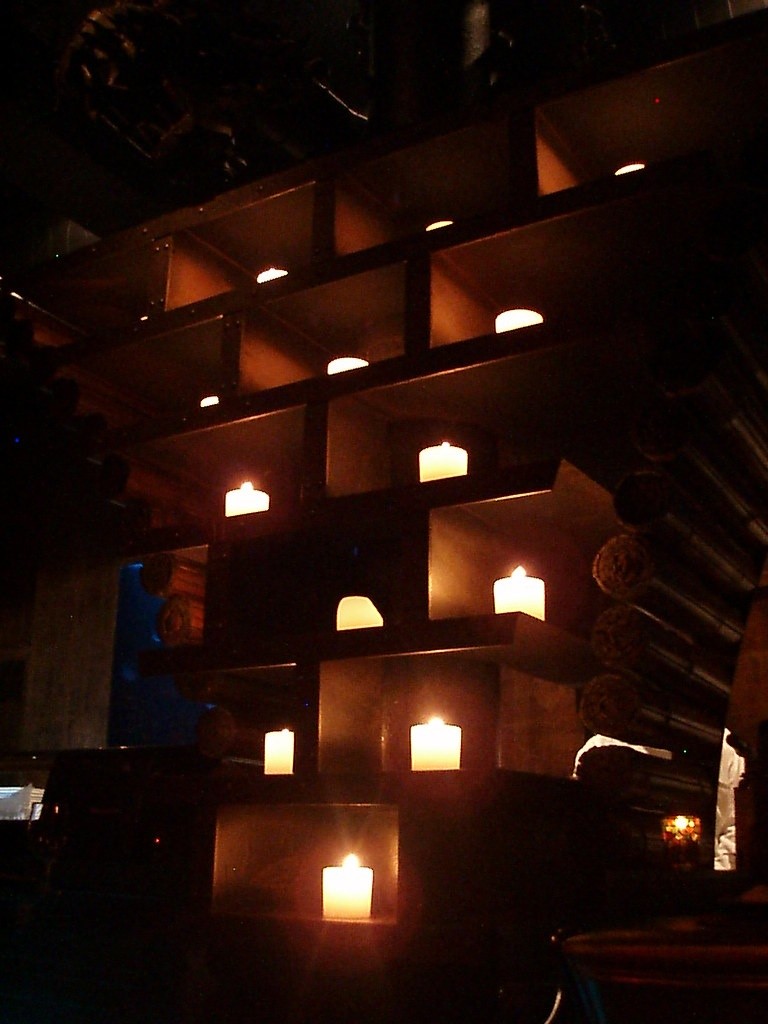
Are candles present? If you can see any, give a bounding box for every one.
[494,565,545,621]
[257,269,288,283]
[329,358,368,376]
[336,597,384,631]
[264,729,294,775]
[495,309,544,334]
[323,853,374,922]
[226,480,269,517]
[419,441,468,481]
[412,716,462,770]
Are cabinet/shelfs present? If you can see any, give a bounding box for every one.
[0,0,768,1024]
